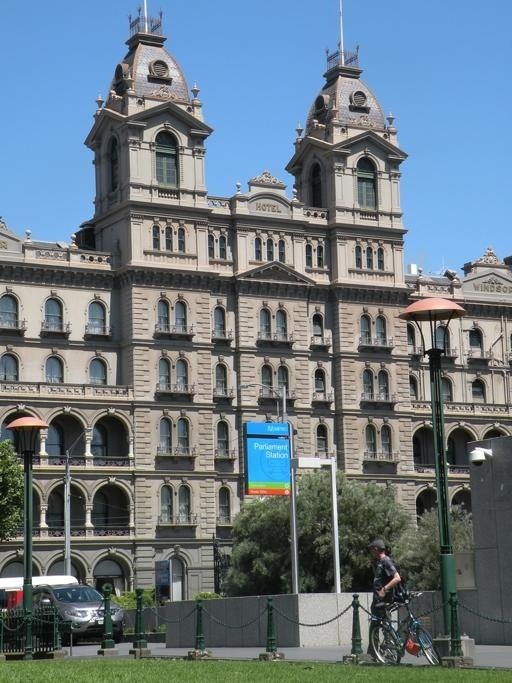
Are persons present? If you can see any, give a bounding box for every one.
[363,537,403,663]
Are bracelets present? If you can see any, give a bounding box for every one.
[383,586,388,592]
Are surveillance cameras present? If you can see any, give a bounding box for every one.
[470,446,486,467]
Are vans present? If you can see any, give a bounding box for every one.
[1,575,78,611]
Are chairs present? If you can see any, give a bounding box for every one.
[59,590,84,602]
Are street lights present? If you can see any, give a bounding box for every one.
[399,298,468,655]
[6,417,49,659]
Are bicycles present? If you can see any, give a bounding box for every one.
[369,592,442,666]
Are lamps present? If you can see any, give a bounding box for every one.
[468,447,494,467]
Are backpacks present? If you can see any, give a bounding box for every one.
[384,575,410,603]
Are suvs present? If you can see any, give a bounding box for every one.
[32,584,123,643]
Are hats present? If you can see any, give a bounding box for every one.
[367,539,386,550]
